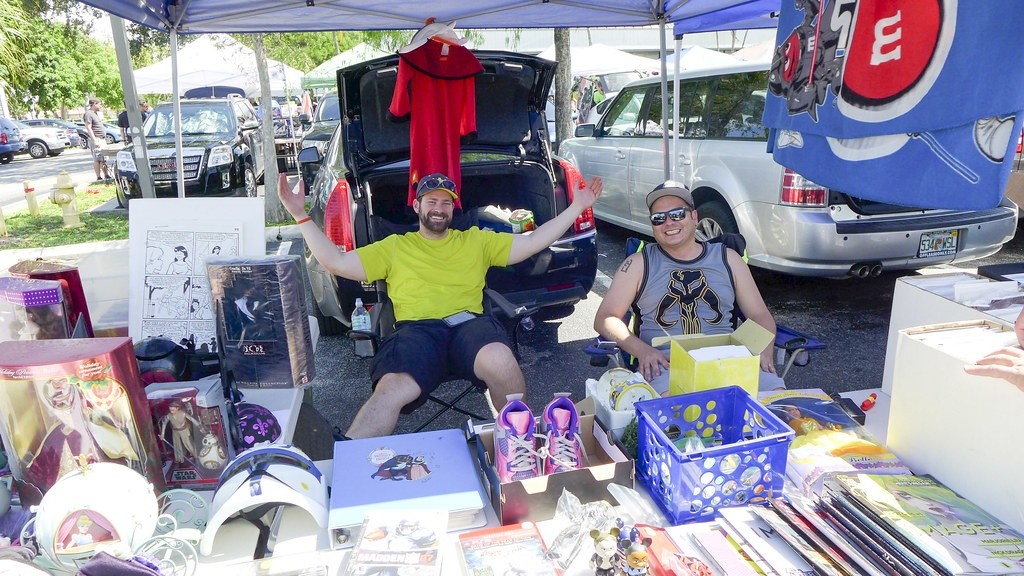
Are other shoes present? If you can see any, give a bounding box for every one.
[97,177,102,180]
[105,175,111,178]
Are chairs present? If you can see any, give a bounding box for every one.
[584,233,829,380]
[669,92,703,137]
[369,207,539,433]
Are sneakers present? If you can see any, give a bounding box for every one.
[539,392,584,477]
[492,393,539,484]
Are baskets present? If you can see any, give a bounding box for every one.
[633,386,795,526]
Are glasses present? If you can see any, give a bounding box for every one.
[649,207,692,225]
[417,178,456,192]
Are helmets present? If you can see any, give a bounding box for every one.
[131,337,191,381]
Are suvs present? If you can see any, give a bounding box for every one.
[112,86,265,208]
[559,58,1018,281]
[298,89,340,195]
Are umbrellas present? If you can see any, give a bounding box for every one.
[120,32,397,102]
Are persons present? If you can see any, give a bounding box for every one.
[117,98,153,145]
[782,404,837,437]
[964,307,1024,396]
[277,174,602,439]
[83,98,112,180]
[589,518,651,576]
[594,180,787,396]
[11,304,66,341]
[160,403,208,465]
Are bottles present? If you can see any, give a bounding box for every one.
[351,298,375,357]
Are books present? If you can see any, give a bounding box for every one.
[691,473,1024,576]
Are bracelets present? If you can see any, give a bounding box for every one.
[297,216,312,224]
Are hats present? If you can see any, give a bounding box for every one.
[646,180,694,211]
[417,173,458,200]
[87,99,101,106]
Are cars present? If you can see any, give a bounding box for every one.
[0,115,128,164]
[302,49,599,336]
[545,66,648,153]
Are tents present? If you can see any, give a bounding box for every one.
[533,41,778,104]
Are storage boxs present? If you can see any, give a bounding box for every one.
[8,261,95,341]
[463,395,635,526]
[0,276,70,339]
[886,318,1024,534]
[144,378,237,490]
[636,384,796,525]
[978,262,1024,287]
[652,318,776,437]
[0,338,171,514]
[880,272,1024,397]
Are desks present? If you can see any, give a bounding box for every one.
[145,316,891,575]
